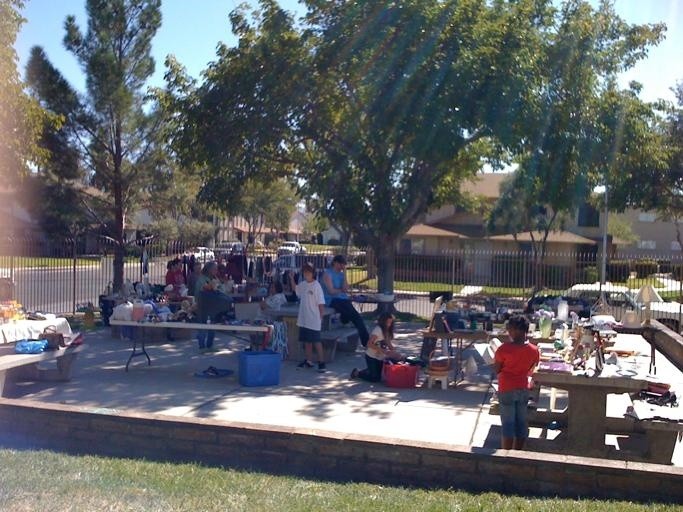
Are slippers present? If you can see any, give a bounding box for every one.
[351,368,359,379]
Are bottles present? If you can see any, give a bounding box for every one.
[557,300,569,322]
[132,298,144,321]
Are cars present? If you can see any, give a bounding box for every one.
[446,285,507,323]
[178,241,335,285]
[523,284,681,333]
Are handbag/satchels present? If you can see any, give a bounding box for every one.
[38,324,65,350]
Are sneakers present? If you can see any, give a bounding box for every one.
[298,359,315,367]
[318,362,326,373]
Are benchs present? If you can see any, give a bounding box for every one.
[1,342,89,394]
[628,389,681,464]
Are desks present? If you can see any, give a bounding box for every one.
[0,318,72,348]
[417,308,645,456]
[109,287,400,373]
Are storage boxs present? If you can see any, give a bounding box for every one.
[383,361,421,388]
[239,350,281,387]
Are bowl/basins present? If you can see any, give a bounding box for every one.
[375,293,394,303]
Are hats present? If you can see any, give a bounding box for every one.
[334,255,347,265]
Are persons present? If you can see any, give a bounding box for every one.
[494,315,540,449]
[286,261,326,373]
[166,257,224,354]
[320,255,369,347]
[348,313,405,382]
[254,280,286,320]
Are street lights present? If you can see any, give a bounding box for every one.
[594,176,609,306]
